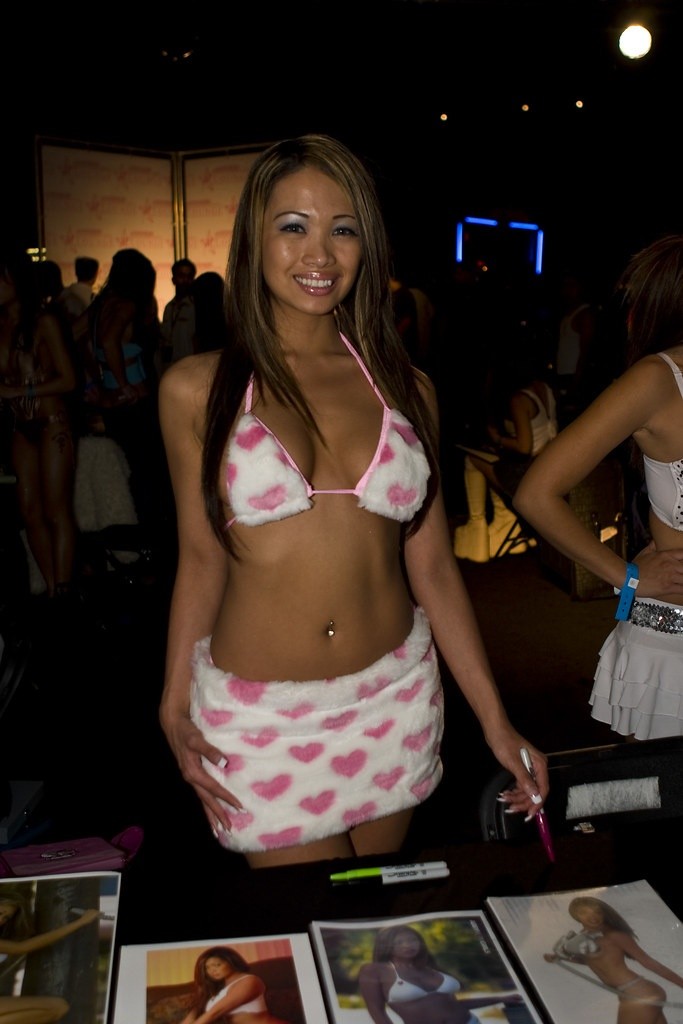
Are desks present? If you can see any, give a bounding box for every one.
[101,818,683,1024]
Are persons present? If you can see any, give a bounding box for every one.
[0,890,104,1024]
[159,133,551,869]
[0,247,226,611]
[513,340,682,744]
[179,947,289,1024]
[378,236,683,565]
[359,925,481,1024]
[544,897,683,1024]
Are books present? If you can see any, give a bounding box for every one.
[0,871,122,1024]
[113,932,329,1024]
[309,909,545,1024]
[483,879,683,1024]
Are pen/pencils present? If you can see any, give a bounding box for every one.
[325,859,451,883]
[520,745,557,869]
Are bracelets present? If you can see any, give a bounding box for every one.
[613,563,639,622]
[28,385,32,398]
